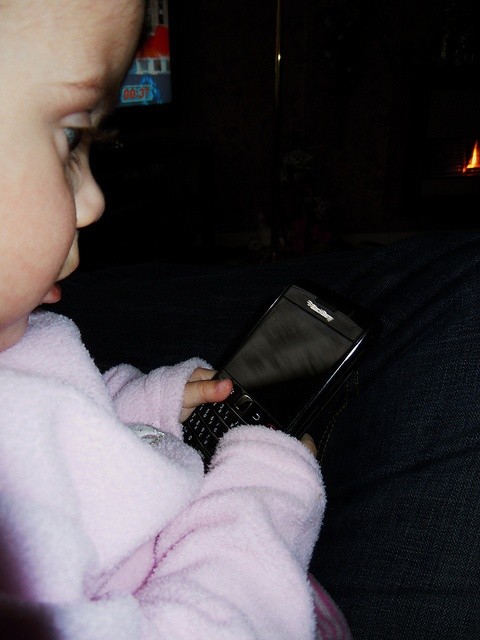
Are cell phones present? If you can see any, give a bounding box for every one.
[181,283,369,475]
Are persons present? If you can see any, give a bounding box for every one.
[1,1,348,638]
